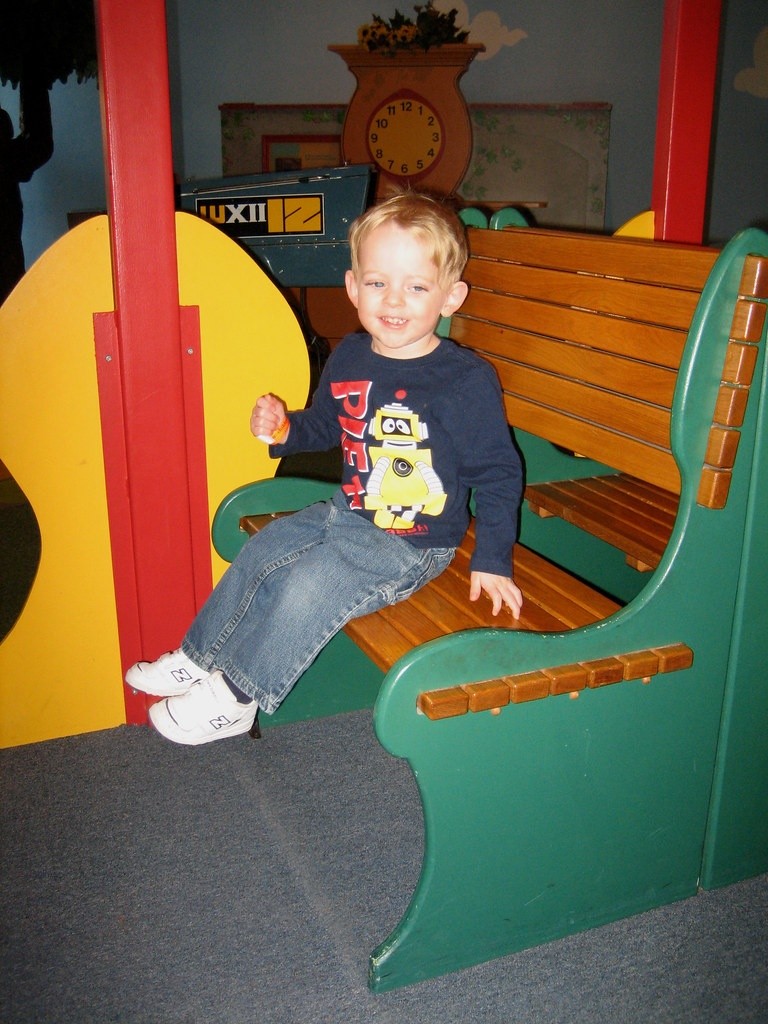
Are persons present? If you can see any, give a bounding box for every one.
[125,186,527,747]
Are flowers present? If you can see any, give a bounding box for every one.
[353,0,471,56]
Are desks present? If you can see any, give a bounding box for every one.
[66,200,548,407]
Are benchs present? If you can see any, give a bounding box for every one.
[211,203,768,996]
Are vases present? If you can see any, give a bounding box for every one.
[326,43,486,203]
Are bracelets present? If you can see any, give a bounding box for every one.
[270,415,290,445]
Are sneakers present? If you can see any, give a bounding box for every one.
[148,669,259,746]
[124,646,212,697]
